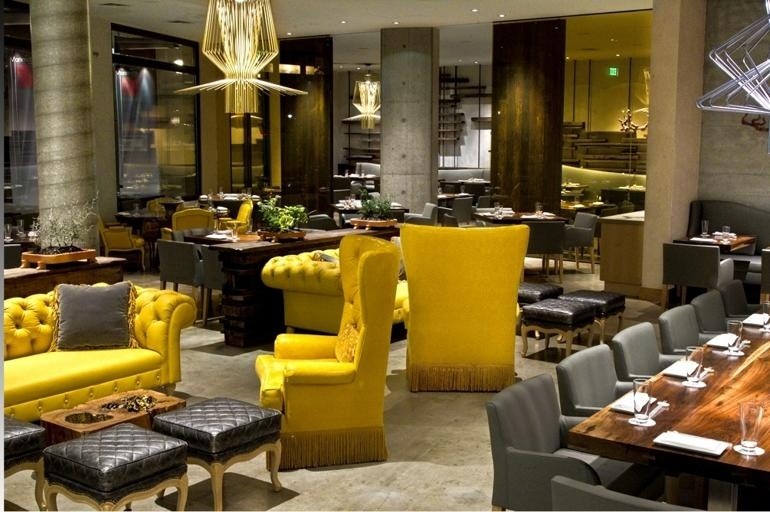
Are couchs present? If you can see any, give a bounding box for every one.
[689,198,769,284]
[261,249,410,334]
[1,281,198,424]
[562,164,646,248]
[355,162,490,187]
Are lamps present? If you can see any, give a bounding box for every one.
[339,75,382,129]
[173,0,310,116]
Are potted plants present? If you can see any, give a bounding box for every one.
[350,187,397,230]
[257,194,316,242]
[20,191,102,270]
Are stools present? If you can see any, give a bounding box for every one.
[151,397,284,511]
[4,412,48,512]
[519,298,601,359]
[516,281,563,339]
[40,421,189,512]
[557,290,624,345]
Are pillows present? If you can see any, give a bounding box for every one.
[390,234,407,277]
[51,279,135,354]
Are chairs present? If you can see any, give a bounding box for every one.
[438,177,446,185]
[200,244,227,326]
[484,372,634,511]
[439,197,473,224]
[658,305,718,357]
[333,188,351,204]
[254,236,403,473]
[565,211,599,276]
[549,475,698,512]
[156,239,212,323]
[612,322,687,380]
[690,287,742,335]
[554,342,644,417]
[3,243,20,269]
[172,230,186,242]
[662,241,734,304]
[442,179,461,194]
[561,199,604,212]
[192,227,213,237]
[146,196,185,212]
[215,197,253,236]
[520,220,567,284]
[471,196,492,216]
[464,182,486,203]
[442,214,459,229]
[97,214,147,272]
[298,215,338,231]
[161,208,215,291]
[593,203,619,257]
[399,223,530,392]
[619,200,635,214]
[715,279,763,318]
[403,202,439,226]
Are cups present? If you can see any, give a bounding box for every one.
[722,225,731,244]
[350,194,355,203]
[762,303,770,330]
[133,203,140,217]
[740,402,764,451]
[242,188,247,194]
[701,220,709,236]
[497,204,504,220]
[247,187,252,196]
[208,189,213,201]
[685,346,705,385]
[218,187,224,197]
[344,196,350,207]
[633,378,650,424]
[535,202,543,218]
[5,224,12,240]
[17,219,25,234]
[726,319,743,355]
